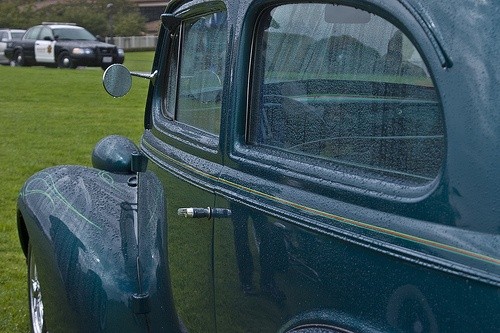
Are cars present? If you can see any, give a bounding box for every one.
[16,0,500,333]
[4,22,125,71]
[0,28,26,66]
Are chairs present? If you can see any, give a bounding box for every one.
[258,95,335,149]
